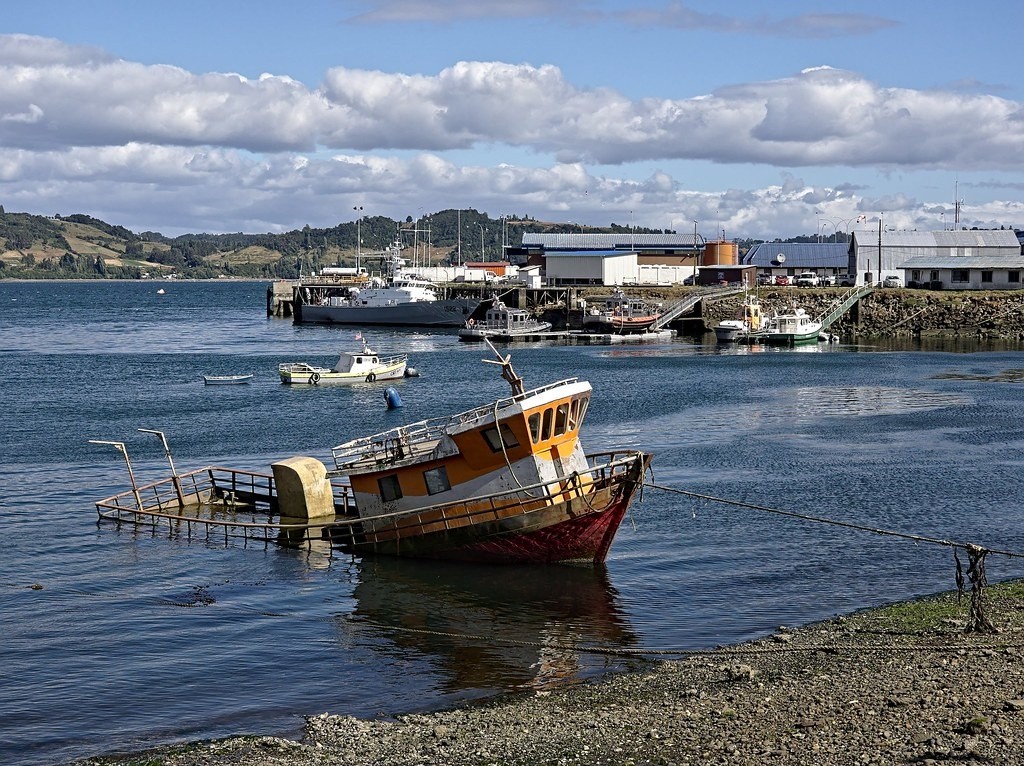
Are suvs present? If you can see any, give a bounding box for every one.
[404,273,431,282]
[798,271,817,287]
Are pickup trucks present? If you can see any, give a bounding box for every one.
[837,274,857,287]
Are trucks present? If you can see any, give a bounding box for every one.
[464,269,499,284]
[321,267,367,277]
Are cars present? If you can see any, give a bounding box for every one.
[885,276,903,287]
[792,275,802,285]
[818,276,830,287]
[492,274,519,284]
[775,275,789,286]
[757,274,772,285]
[683,274,699,286]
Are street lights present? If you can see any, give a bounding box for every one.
[415,206,433,267]
[821,216,858,243]
[941,212,947,231]
[816,211,819,243]
[501,216,509,261]
[694,220,698,285]
[353,206,363,275]
[568,221,583,234]
[458,208,465,267]
[881,212,883,230]
[474,221,484,263]
[821,224,827,244]
[716,210,720,264]
[631,210,633,252]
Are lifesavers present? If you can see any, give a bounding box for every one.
[311,373,320,382]
[743,319,750,327]
[469,319,474,324]
[368,372,376,382]
[616,306,620,312]
[280,375,287,383]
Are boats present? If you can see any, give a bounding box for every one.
[716,273,770,346]
[277,337,408,385]
[582,291,665,334]
[89,336,649,582]
[202,374,254,385]
[456,302,552,341]
[300,231,485,329]
[735,302,823,346]
[817,332,840,343]
